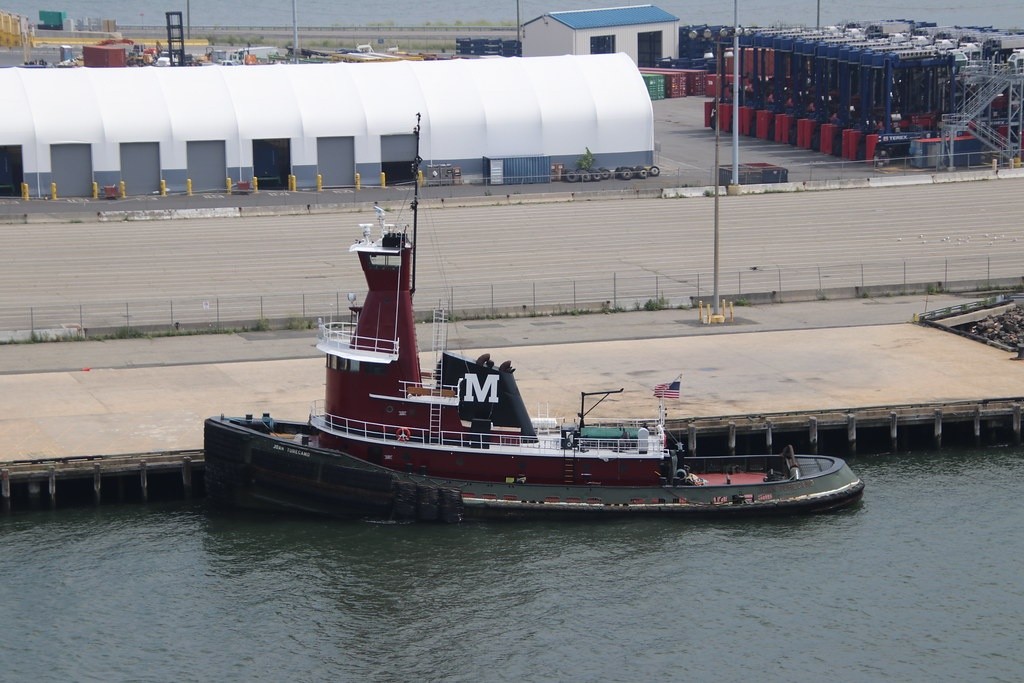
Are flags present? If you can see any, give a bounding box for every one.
[654,382,679,398]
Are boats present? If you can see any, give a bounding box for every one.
[202,109,867,525]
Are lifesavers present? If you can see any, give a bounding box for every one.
[396,428,411,441]
